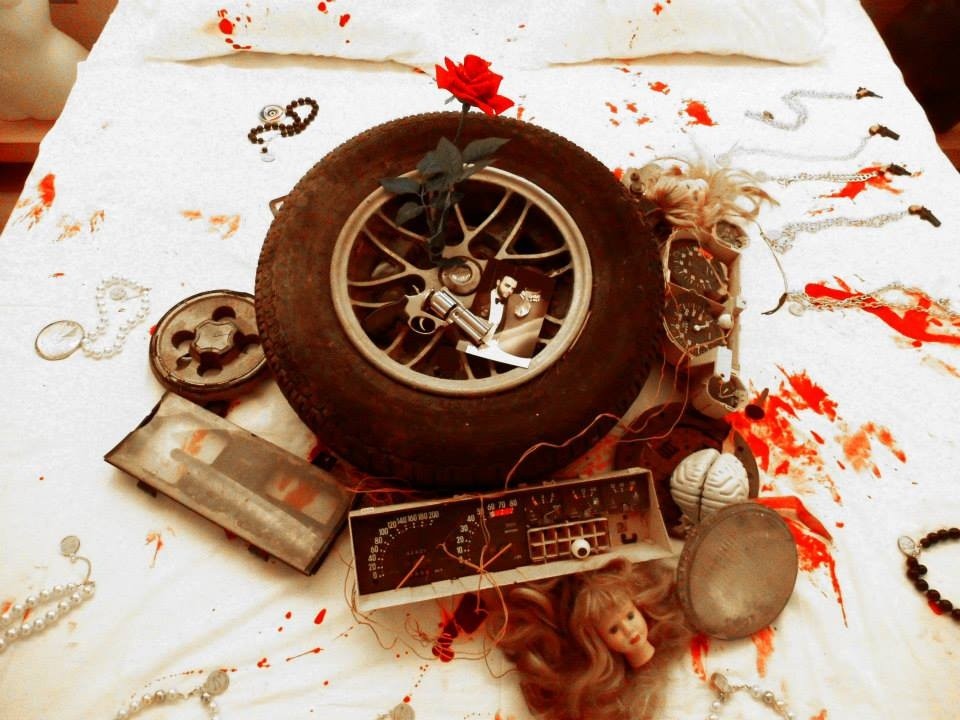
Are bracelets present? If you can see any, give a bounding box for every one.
[248,98,319,163]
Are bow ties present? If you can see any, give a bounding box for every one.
[495,298,505,304]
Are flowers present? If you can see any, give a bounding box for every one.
[386,40,517,252]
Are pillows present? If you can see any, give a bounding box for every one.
[135,0,848,63]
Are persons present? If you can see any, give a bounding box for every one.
[645,166,762,231]
[495,556,691,720]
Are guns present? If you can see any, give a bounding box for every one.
[361,283,496,345]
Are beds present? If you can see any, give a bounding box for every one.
[0,0,960,720]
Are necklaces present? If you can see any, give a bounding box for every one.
[116,670,229,720]
[897,527,960,621]
[745,87,883,132]
[785,282,957,322]
[760,205,941,256]
[0,537,95,652]
[709,672,795,720]
[35,275,149,360]
[737,123,899,162]
[775,163,912,189]
